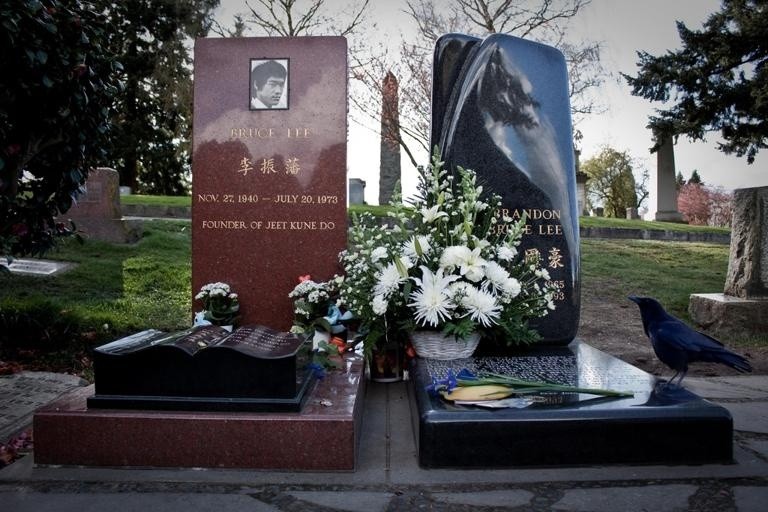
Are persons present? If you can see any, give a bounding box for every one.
[250,60,287,109]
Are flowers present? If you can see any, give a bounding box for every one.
[425,368,635,401]
[193,280,241,326]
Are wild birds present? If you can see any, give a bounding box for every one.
[625,292,752,393]
